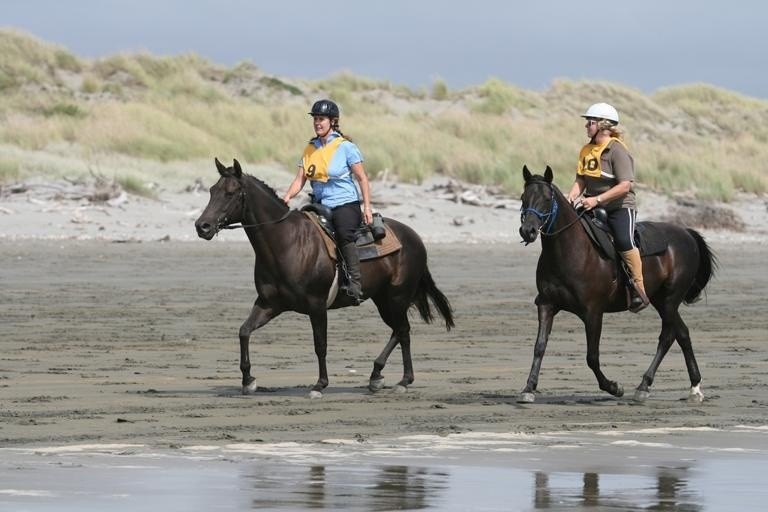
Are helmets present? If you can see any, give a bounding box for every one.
[580,103,619,125]
[308,100,339,118]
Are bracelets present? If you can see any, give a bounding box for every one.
[595,194,602,207]
[364,207,372,210]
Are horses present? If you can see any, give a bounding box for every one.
[193,156,457,400]
[515,164,719,405]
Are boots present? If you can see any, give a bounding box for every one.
[340,241,362,306]
[619,246,647,307]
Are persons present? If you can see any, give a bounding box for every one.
[566,103,649,312]
[281,99,375,307]
